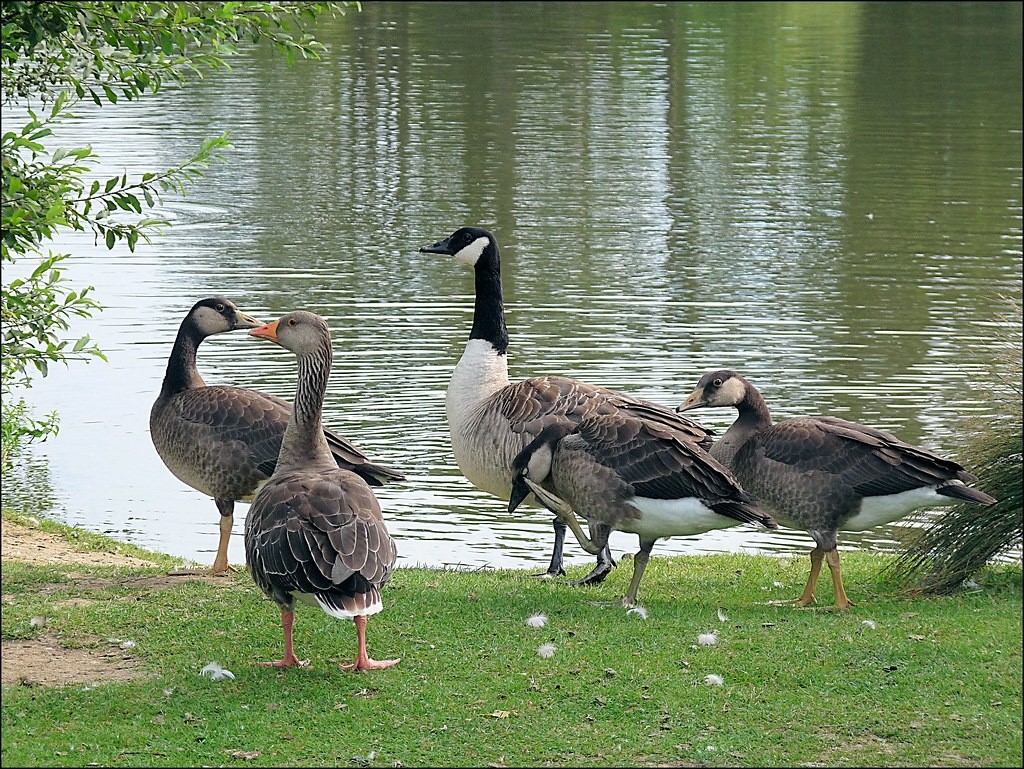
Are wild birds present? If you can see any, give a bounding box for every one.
[148,297,404,673]
[417,226,998,613]
